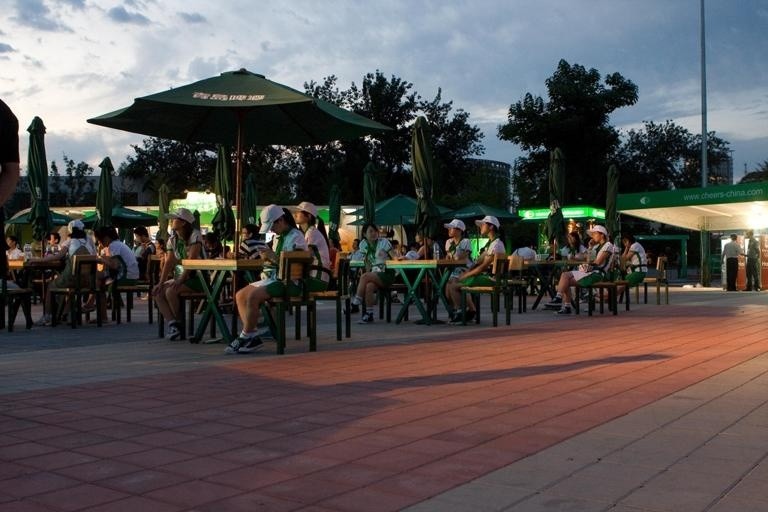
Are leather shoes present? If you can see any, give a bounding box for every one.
[742,287,752,291]
[756,288,761,292]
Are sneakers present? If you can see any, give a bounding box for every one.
[81,305,95,313]
[581,287,596,303]
[342,303,360,317]
[558,307,573,316]
[357,312,374,324]
[447,306,476,325]
[546,297,563,307]
[223,316,270,354]
[35,318,52,326]
[166,321,181,340]
[88,319,112,327]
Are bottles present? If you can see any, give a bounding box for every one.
[54,242,58,256]
[46,242,51,257]
[24,242,30,261]
[433,242,439,260]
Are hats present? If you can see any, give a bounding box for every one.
[164,208,196,224]
[475,216,501,230]
[585,225,608,239]
[259,204,284,234]
[406,251,421,260]
[444,218,467,232]
[291,202,319,219]
[68,219,86,234]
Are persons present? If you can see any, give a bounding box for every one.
[1,204,647,353]
[722,230,761,291]
